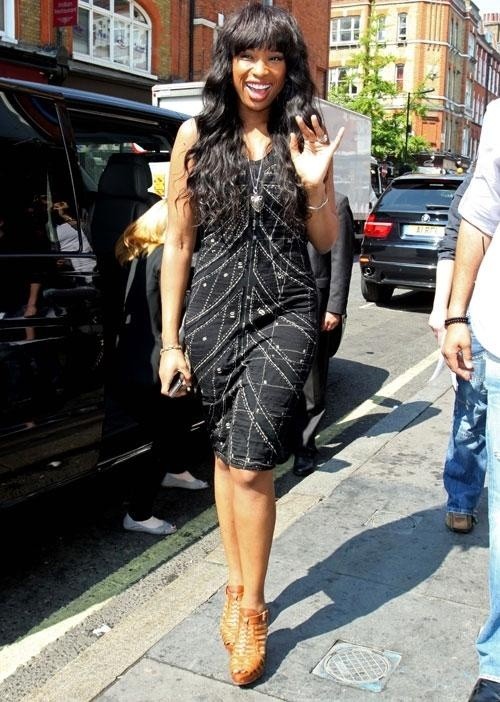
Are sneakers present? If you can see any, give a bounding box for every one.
[163,471,209,489]
[446,511,477,533]
[124,513,177,534]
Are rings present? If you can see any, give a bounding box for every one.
[316,135,329,145]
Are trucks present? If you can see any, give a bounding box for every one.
[142,78,384,251]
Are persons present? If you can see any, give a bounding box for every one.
[441,98,499,701]
[18,195,99,342]
[157,2,346,688]
[290,192,355,476]
[426,154,489,534]
[97,192,213,536]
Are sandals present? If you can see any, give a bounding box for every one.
[230,607,269,685]
[220,585,243,651]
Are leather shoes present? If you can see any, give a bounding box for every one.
[468,679,499,701]
[294,449,317,476]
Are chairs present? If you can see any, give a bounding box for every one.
[92,153,161,341]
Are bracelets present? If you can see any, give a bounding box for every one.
[445,317,469,326]
[159,346,187,355]
[302,200,332,210]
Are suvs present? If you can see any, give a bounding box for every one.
[356,169,471,303]
[0,26,346,507]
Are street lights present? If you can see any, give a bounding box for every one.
[403,88,438,160]
